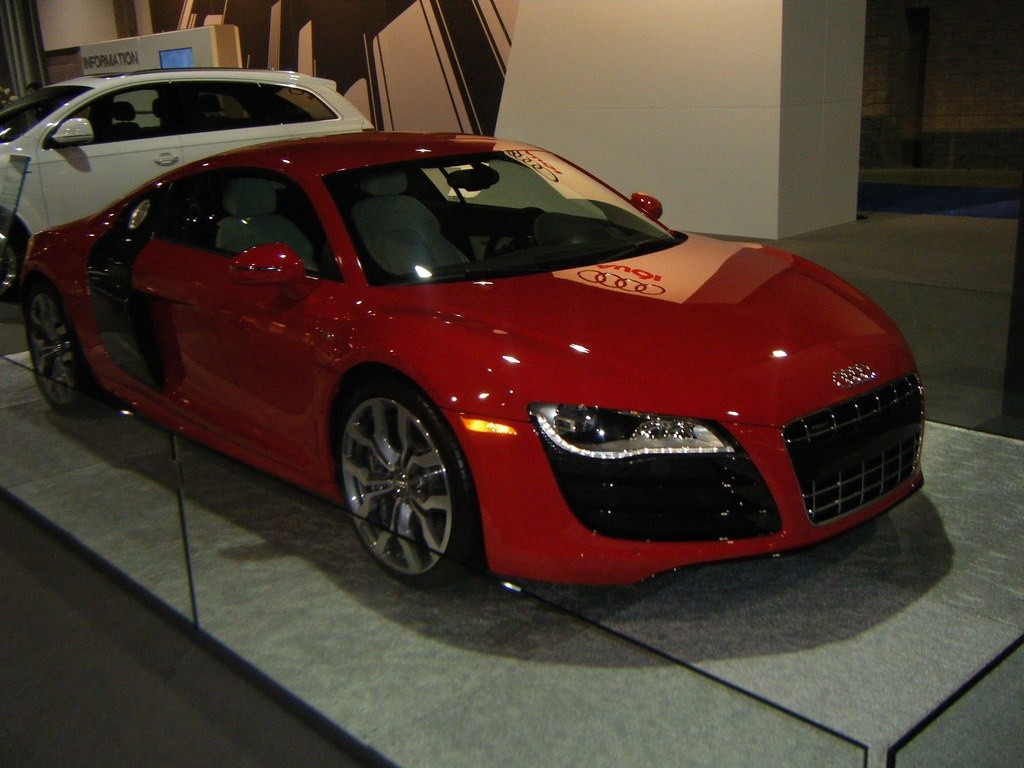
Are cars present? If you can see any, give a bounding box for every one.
[0,66,378,303]
[17,131,926,590]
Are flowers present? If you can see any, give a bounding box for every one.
[0,88,19,107]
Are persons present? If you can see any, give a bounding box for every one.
[25,82,59,122]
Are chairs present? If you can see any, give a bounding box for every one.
[110,102,139,131]
[201,94,232,126]
[215,178,317,274]
[352,174,469,280]
